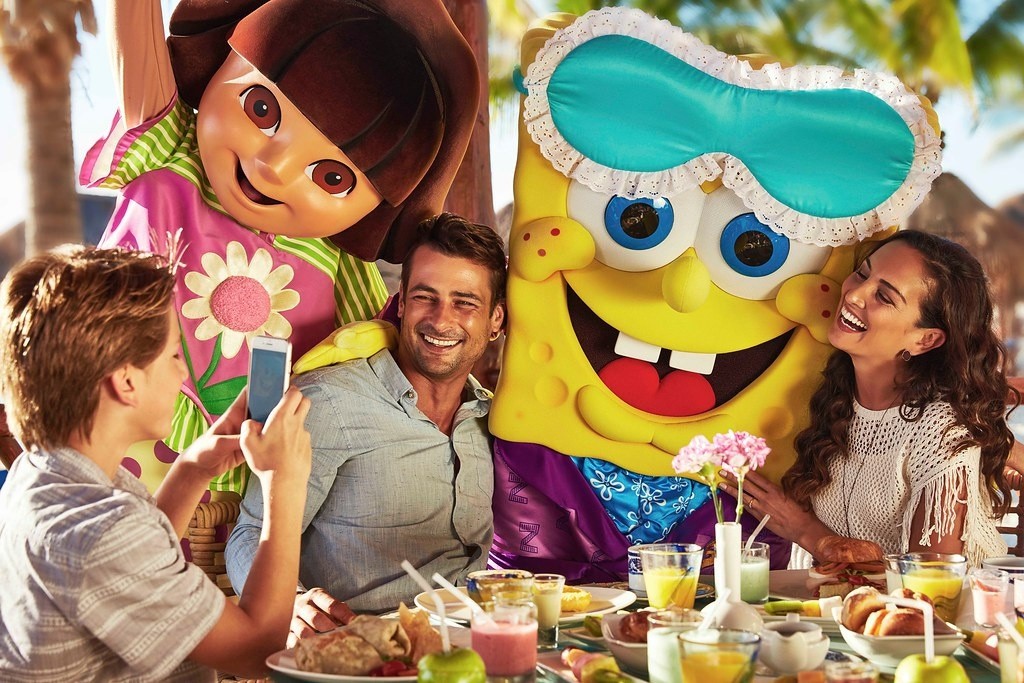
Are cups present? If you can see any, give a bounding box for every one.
[995,609,1024,683]
[882,551,969,626]
[626,545,667,591]
[969,569,1010,630]
[528,574,566,650]
[676,625,763,683]
[639,543,704,610]
[466,571,537,683]
[713,541,770,609]
[645,610,706,683]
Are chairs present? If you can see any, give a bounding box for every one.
[187,502,275,683]
[988,438,1023,559]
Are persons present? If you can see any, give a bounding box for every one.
[76,0,479,588]
[0,243,313,683]
[717,226,1019,570]
[223,211,495,643]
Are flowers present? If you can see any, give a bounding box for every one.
[671,430,772,525]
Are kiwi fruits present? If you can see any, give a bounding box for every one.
[763,600,804,616]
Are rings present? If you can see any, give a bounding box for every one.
[749,498,754,509]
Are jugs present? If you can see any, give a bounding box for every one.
[754,612,831,673]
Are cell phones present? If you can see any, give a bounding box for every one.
[244,336,292,427]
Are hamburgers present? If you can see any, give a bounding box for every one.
[806,536,889,596]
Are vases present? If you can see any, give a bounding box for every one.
[700,522,764,631]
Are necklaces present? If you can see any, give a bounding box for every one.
[842,391,904,538]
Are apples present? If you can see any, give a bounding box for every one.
[892,652,971,683]
[415,646,488,683]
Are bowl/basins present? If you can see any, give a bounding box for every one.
[601,613,647,676]
[982,557,1024,582]
[832,605,968,673]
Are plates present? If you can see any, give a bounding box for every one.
[414,583,637,625]
[755,648,879,683]
[265,646,419,683]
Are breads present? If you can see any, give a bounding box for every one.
[840,587,954,637]
[620,609,663,644]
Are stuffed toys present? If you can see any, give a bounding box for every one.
[487,8,943,586]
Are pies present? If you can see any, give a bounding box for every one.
[294,602,448,677]
